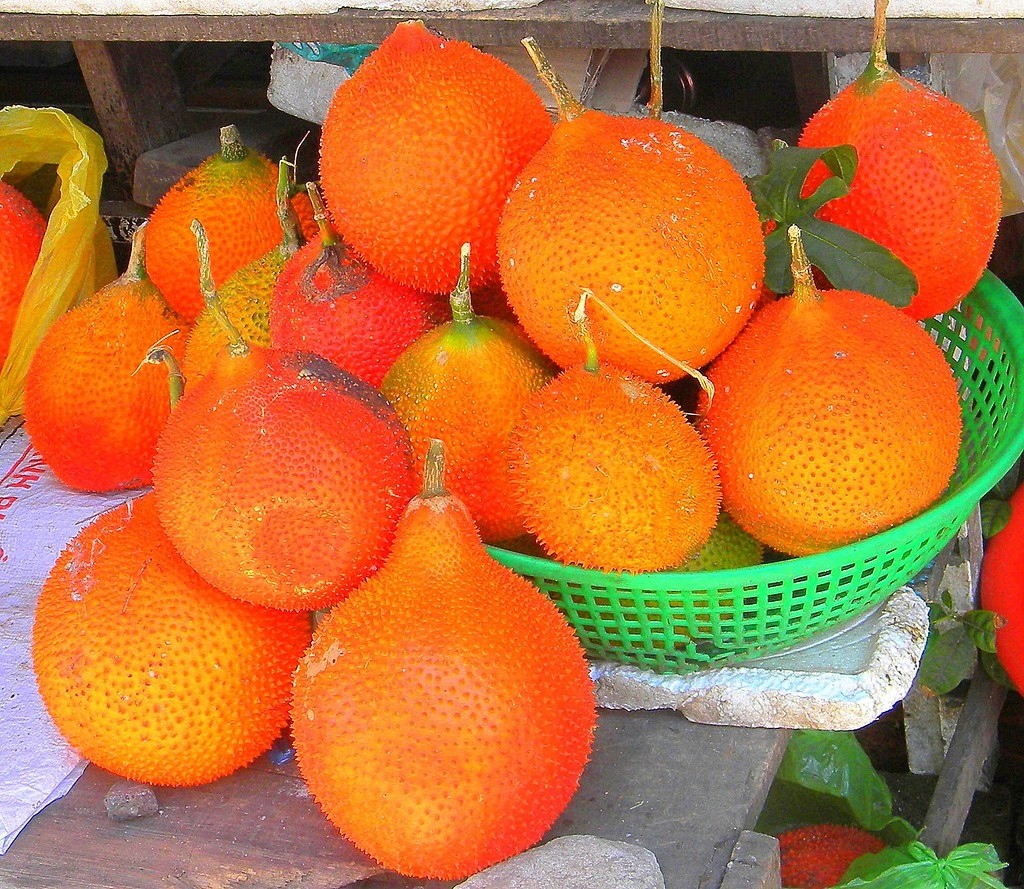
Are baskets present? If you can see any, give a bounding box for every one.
[484,267,1024,668]
